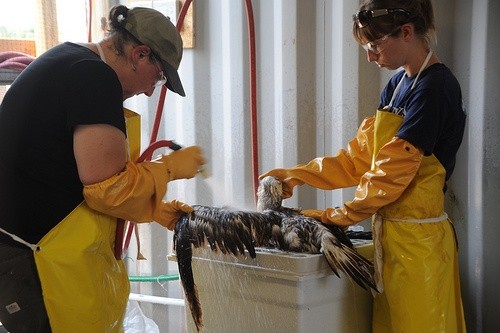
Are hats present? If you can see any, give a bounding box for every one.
[117,6,185,97]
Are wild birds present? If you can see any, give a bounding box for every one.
[169,171,383,333]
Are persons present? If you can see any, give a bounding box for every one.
[256,0,467,333]
[0,4,206,332]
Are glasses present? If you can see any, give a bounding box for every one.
[150,51,167,87]
[352,8,410,28]
[361,25,401,55]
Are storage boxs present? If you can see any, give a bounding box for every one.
[166,233,378,333]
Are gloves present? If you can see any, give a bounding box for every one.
[83,146,208,224]
[299,136,424,232]
[256,114,376,200]
[153,199,194,232]
